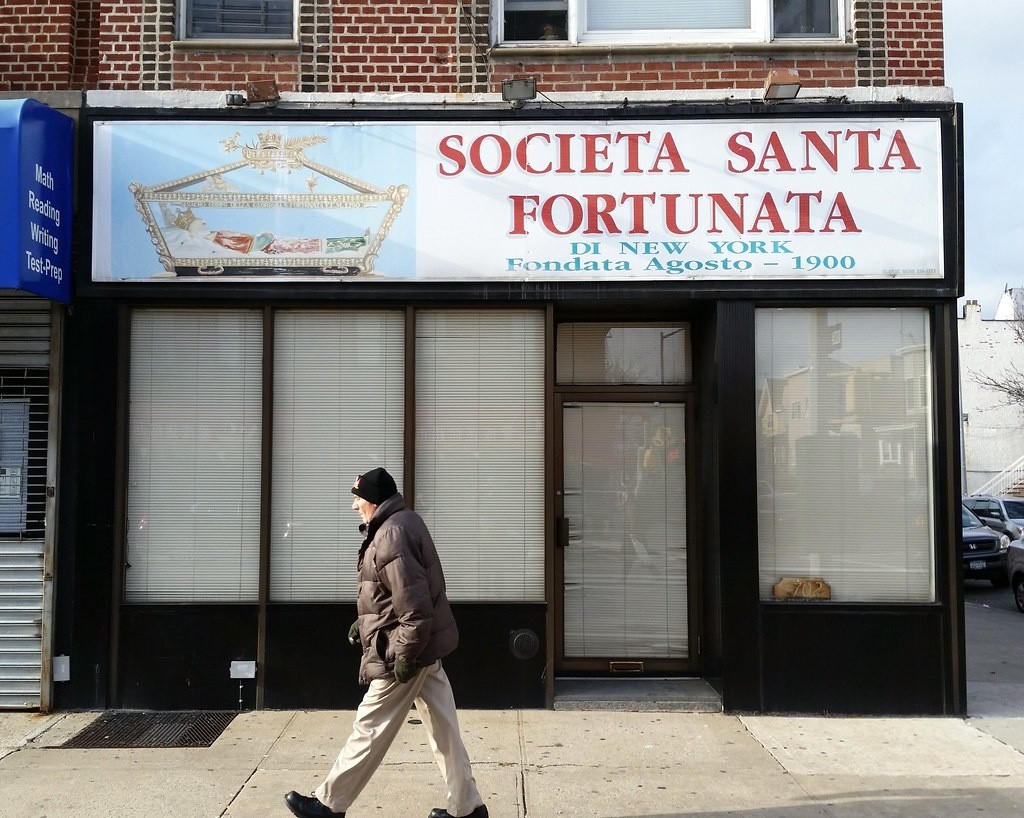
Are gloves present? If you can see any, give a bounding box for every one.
[348,619,360,644]
[394,659,417,683]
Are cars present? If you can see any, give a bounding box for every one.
[137,496,305,567]
[960,505,1010,586]
[1008,527,1024,613]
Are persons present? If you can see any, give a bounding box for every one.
[619,490,659,586]
[283,467,488,818]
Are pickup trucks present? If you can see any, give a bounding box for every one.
[756,481,800,526]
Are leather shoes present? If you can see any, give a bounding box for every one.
[428,804,489,818]
[283,790,346,818]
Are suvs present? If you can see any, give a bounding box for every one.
[962,493,1023,534]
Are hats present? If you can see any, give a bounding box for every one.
[351,467,398,506]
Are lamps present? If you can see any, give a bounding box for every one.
[762,68,802,103]
[501,77,537,108]
